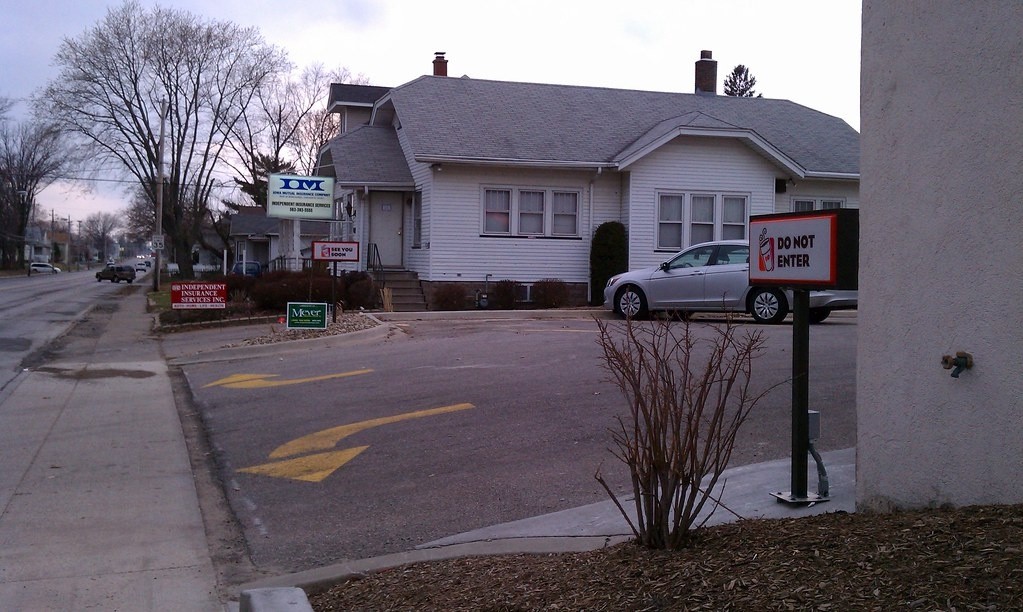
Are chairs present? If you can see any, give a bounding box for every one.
[718,254,730,264]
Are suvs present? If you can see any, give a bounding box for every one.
[96,264,137,284]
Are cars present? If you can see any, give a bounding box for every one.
[225,261,262,281]
[135,262,146,272]
[106,259,116,267]
[603,239,859,325]
[136,253,151,259]
[144,261,151,268]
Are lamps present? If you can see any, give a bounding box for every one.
[345,200,356,217]
[787,178,797,188]
[433,163,442,171]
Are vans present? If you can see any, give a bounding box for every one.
[30,262,62,275]
